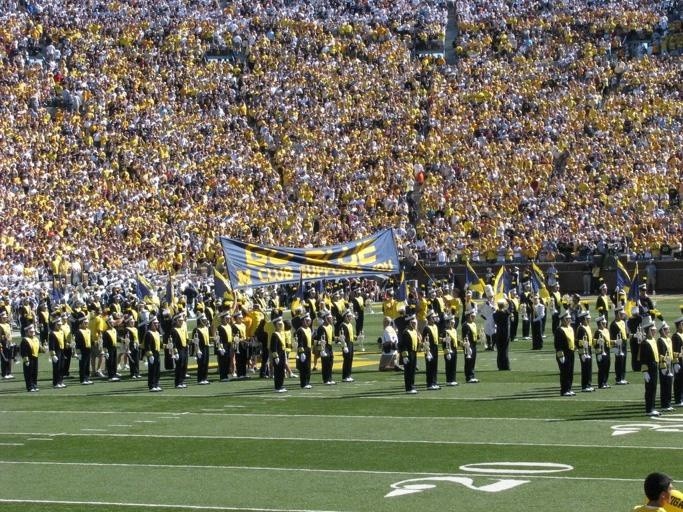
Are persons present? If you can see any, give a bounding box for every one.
[633,473,673,512]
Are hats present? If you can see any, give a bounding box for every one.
[482,265,647,322]
[0,286,362,332]
[673,317,683,326]
[657,321,670,331]
[643,321,657,330]
[386,279,476,323]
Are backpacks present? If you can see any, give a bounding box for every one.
[616,339,622,346]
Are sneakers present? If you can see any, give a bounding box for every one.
[646,402,683,416]
[561,379,630,397]
[28,370,144,392]
[4,374,15,380]
[176,365,271,389]
[150,387,163,392]
[512,338,518,342]
[273,364,355,394]
[523,337,532,340]
[406,379,480,395]
[542,335,546,338]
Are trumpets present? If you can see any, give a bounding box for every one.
[583,331,624,359]
[294,331,365,359]
[549,297,555,315]
[665,351,674,376]
[519,303,528,320]
[424,333,472,359]
[168,331,240,358]
[70,332,132,358]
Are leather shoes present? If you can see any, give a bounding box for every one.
[394,364,404,372]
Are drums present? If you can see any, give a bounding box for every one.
[250,343,261,355]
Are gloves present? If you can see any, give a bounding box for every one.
[319,350,327,356]
[402,337,472,366]
[275,356,279,365]
[643,373,651,384]
[300,352,306,361]
[634,334,638,338]
[661,368,668,376]
[343,346,348,353]
[583,341,588,348]
[597,339,603,345]
[597,355,603,362]
[22,334,241,368]
[581,355,587,362]
[673,363,681,374]
[559,357,565,364]
